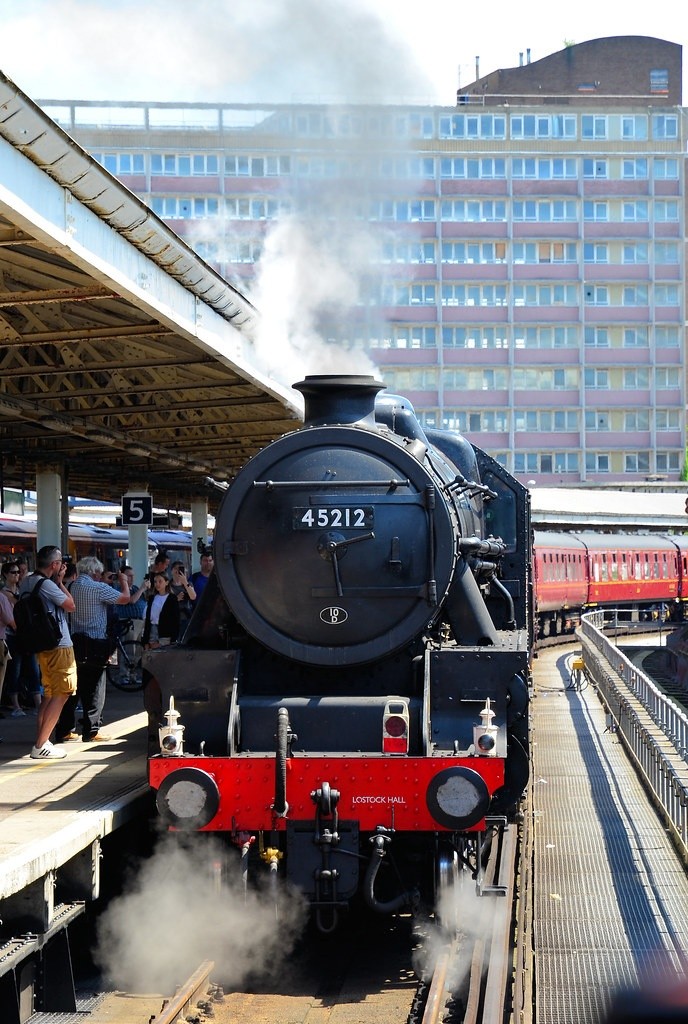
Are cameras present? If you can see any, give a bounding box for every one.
[61,554,72,565]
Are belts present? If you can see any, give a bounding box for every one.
[123,616,142,620]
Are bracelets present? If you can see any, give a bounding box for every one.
[185,584,189,588]
[120,578,128,581]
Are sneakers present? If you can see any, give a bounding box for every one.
[30,708,38,715]
[12,708,26,717]
[83,733,111,742]
[30,739,67,759]
[63,732,78,741]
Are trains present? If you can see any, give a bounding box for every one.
[140,377,687,941]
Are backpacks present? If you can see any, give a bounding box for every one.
[12,578,63,653]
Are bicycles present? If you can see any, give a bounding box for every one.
[102,604,149,693]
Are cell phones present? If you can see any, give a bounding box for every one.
[179,566,184,575]
[111,574,118,581]
[145,574,150,581]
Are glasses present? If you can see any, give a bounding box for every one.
[51,558,64,565]
[10,571,21,574]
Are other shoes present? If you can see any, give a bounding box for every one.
[130,678,142,684]
[120,676,129,684]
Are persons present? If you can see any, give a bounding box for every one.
[0,545,217,759]
